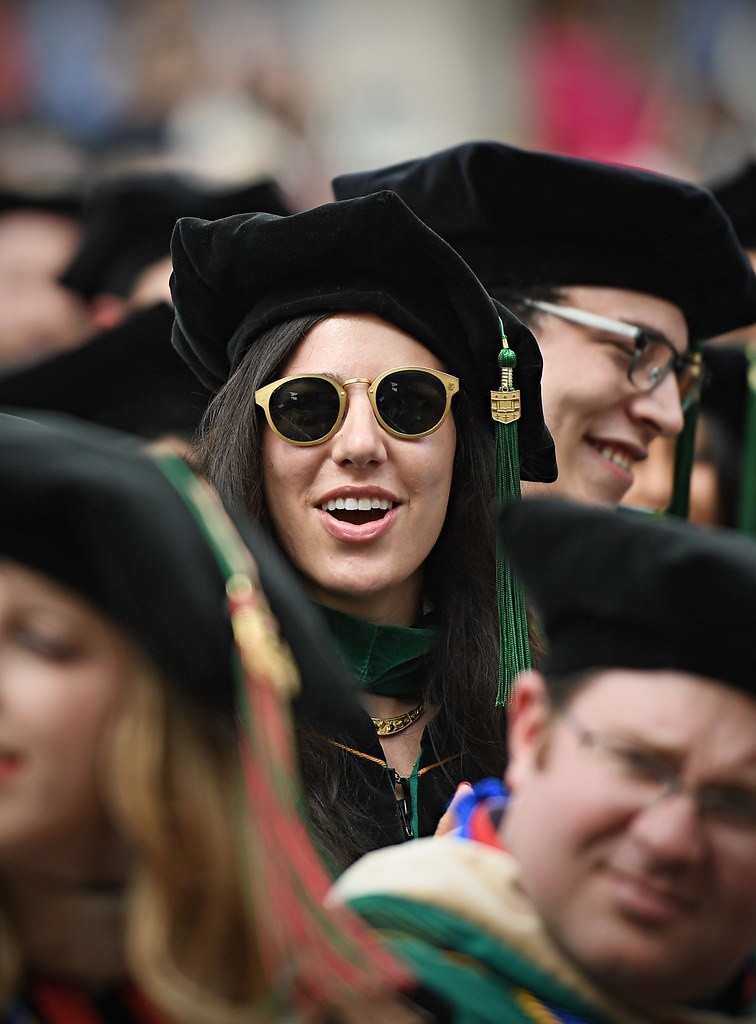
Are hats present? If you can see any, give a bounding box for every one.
[0,409,238,716]
[673,156,755,532]
[170,191,558,706]
[498,498,756,701]
[1,173,294,305]
[330,140,756,345]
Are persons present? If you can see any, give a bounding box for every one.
[168,188,559,881]
[0,411,449,1024]
[0,140,756,529]
[323,490,756,1024]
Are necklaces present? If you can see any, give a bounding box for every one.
[370,702,428,736]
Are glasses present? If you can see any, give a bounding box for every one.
[518,293,711,415]
[251,367,464,447]
[565,707,756,842]
